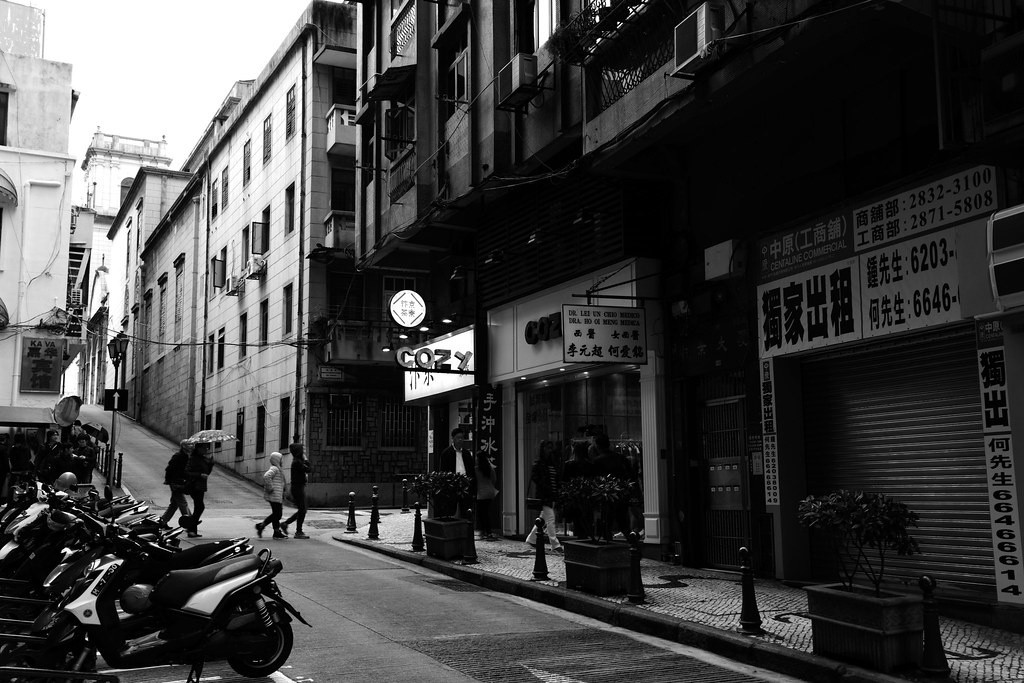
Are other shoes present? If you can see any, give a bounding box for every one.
[187,532,203,538]
[197,520,202,525]
[273,529,288,540]
[255,523,264,538]
[293,530,310,539]
[280,521,288,535]
[553,547,564,555]
[159,520,173,530]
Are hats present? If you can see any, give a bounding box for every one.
[47,430,59,436]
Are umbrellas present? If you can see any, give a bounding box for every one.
[186,430,240,453]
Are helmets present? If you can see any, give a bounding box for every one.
[121,584,154,614]
[54,470,77,492]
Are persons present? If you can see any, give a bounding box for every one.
[526,433,641,552]
[0,427,100,497]
[255,451,288,539]
[156,438,214,538]
[439,428,499,542]
[280,444,313,539]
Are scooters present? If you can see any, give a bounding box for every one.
[1,470,313,683]
